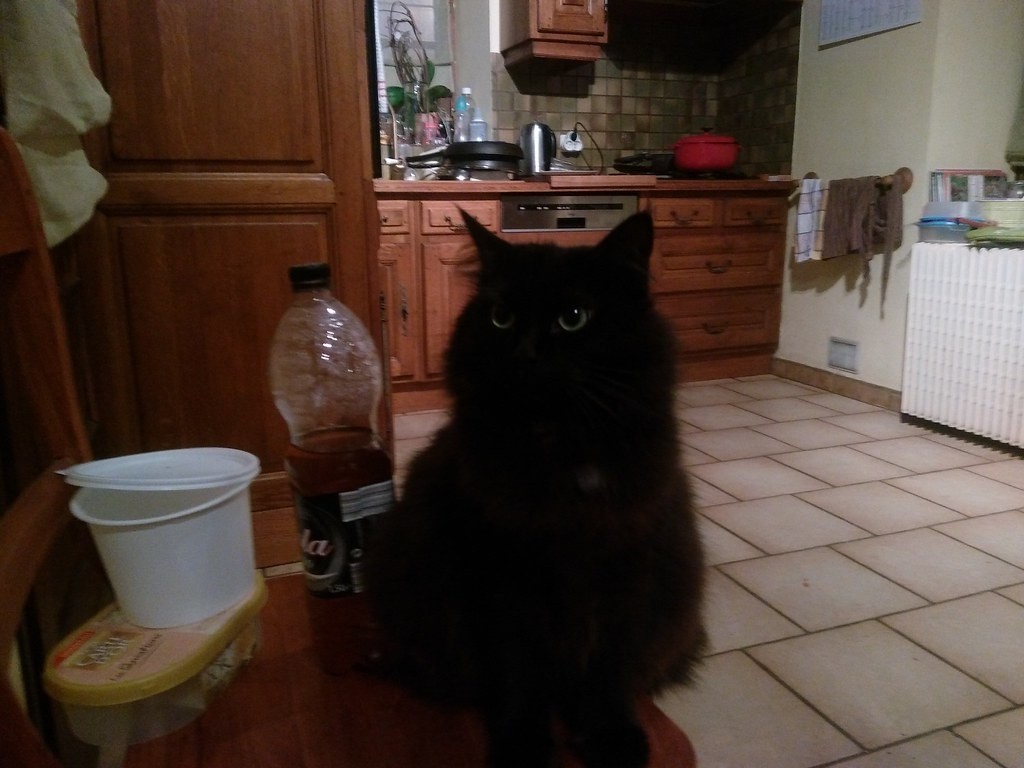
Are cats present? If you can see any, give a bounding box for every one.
[353,206,714,768]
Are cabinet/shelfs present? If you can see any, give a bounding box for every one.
[499,1,610,76]
[377,193,786,414]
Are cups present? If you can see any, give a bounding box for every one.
[403,82,429,136]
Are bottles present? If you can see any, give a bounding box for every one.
[271,262,397,679]
[469,107,486,141]
[454,87,475,142]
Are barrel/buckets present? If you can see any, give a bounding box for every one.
[56,448,262,629]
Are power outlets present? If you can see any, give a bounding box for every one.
[560,131,583,151]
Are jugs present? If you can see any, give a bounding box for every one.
[521,120,556,176]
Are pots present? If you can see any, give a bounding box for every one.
[406,159,522,169]
[668,127,743,169]
[405,142,525,163]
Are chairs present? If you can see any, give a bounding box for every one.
[0,127,698,768]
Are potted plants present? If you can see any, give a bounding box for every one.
[386,61,453,146]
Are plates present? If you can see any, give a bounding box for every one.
[534,171,599,175]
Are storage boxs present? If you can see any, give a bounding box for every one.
[915,221,970,243]
